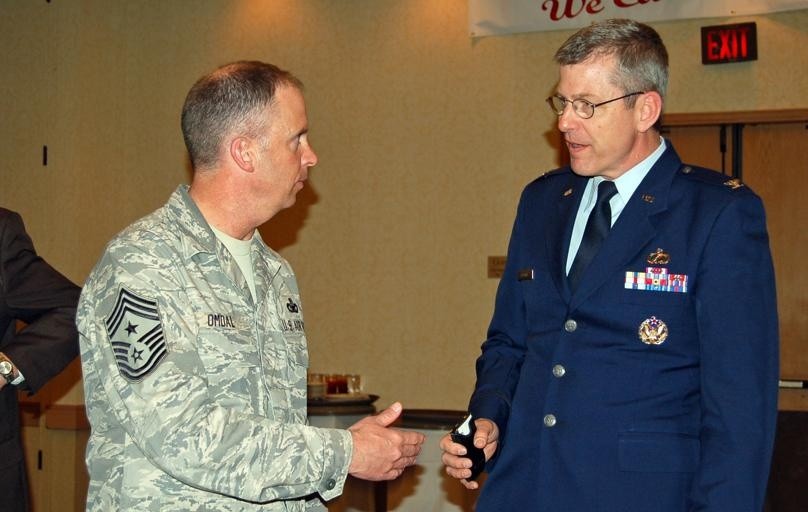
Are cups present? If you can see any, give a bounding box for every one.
[310,373,361,394]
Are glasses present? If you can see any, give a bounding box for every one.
[546,81,644,119]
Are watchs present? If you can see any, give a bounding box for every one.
[1,354,15,384]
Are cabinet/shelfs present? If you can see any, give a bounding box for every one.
[307,403,471,512]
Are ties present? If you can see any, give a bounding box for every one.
[567,181,618,298]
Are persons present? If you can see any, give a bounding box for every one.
[438,18,782,512]
[75,59,426,512]
[1,207,83,511]
